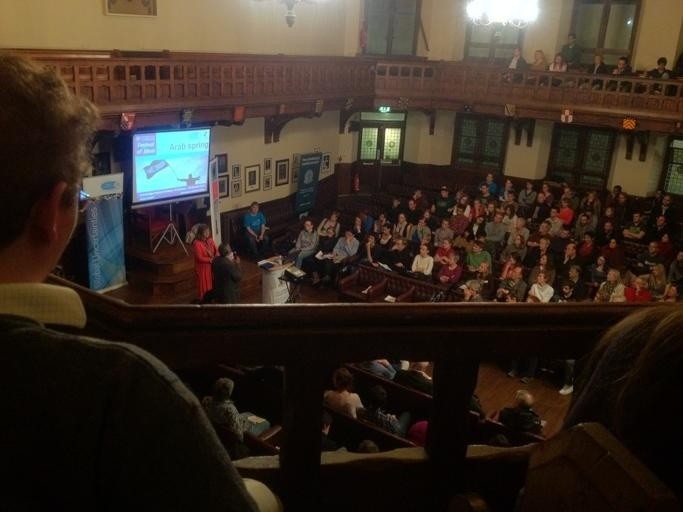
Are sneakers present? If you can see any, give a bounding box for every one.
[507,368,555,384]
[558,383,574,396]
[310,276,333,288]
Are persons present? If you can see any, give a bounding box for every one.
[548,54,567,87]
[527,50,548,87]
[651,56,673,97]
[504,45,526,83]
[519,299,682,510]
[579,55,609,90]
[607,56,632,92]
[0,53,284,512]
[561,32,582,67]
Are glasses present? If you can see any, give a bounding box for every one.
[67,182,97,215]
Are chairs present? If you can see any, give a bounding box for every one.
[221,177,683,303]
[204,341,546,455]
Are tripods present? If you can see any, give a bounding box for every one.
[152,202,190,256]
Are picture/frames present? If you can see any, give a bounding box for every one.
[104,0,158,18]
